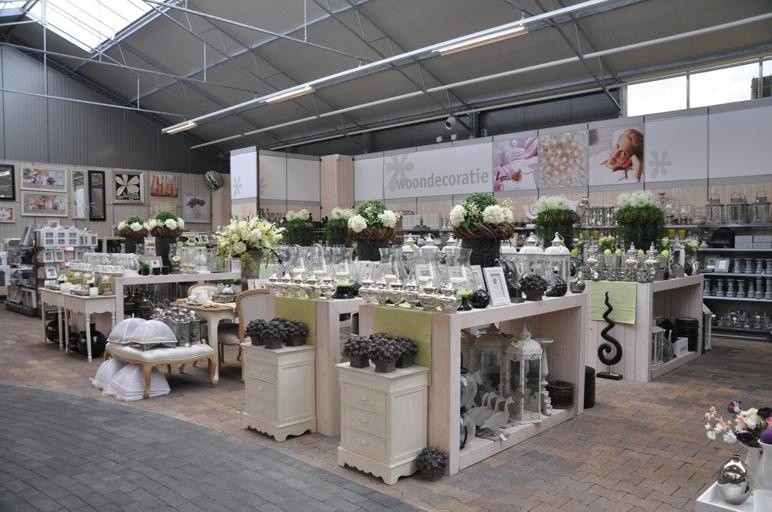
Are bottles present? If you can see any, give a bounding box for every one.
[260,207,287,229]
[149,302,203,351]
[569,229,711,282]
[711,308,772,331]
[583,204,625,228]
[268,232,570,314]
[44,313,105,359]
[653,190,772,225]
[67,240,226,297]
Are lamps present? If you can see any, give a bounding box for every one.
[259,85,316,105]
[444,116,457,130]
[166,121,198,135]
[430,25,529,57]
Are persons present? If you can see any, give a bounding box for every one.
[586,128,644,183]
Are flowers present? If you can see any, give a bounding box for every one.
[533,195,578,240]
[449,193,513,228]
[113,215,149,238]
[613,191,665,248]
[331,199,397,232]
[703,399,762,448]
[211,213,285,263]
[147,211,185,237]
[754,406,772,445]
[285,208,310,221]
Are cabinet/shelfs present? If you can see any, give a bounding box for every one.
[694,462,772,512]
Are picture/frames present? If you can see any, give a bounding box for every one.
[0,163,210,224]
[0,226,98,309]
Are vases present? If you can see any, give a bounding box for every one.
[238,248,261,279]
[351,225,393,261]
[125,236,144,253]
[740,441,760,494]
[154,236,177,265]
[752,439,772,493]
